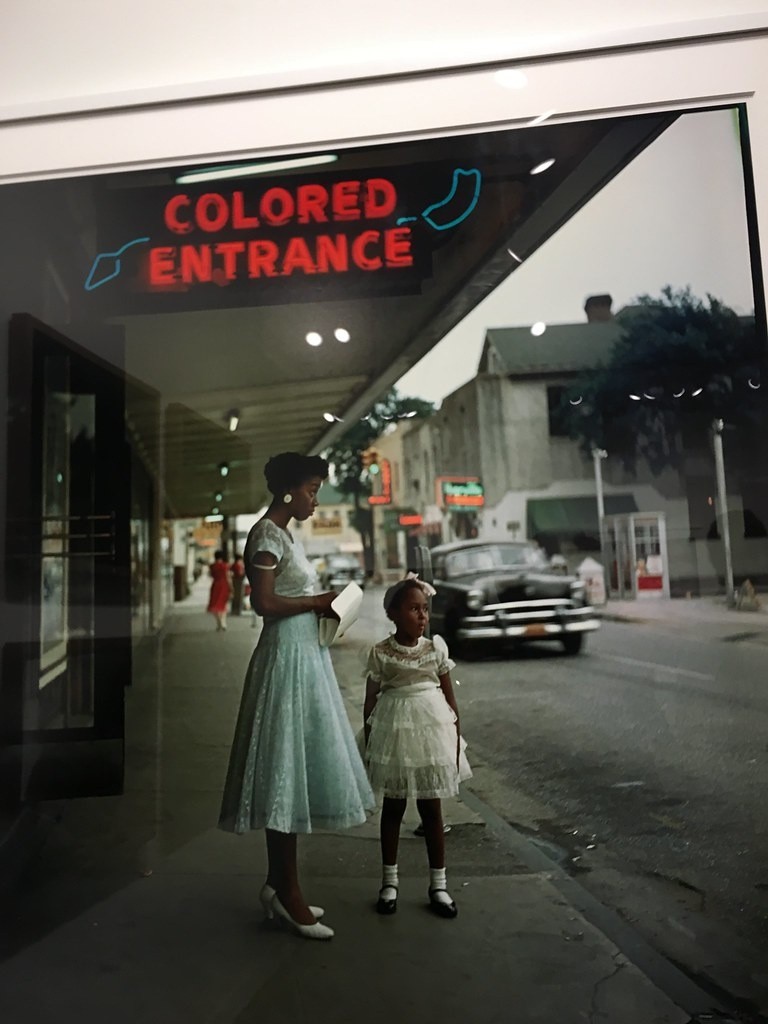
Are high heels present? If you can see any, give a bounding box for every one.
[271,894,335,939]
[260,884,325,919]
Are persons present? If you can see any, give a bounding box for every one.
[218,452,376,938]
[357,579,474,918]
[636,555,649,578]
[207,551,232,630]
[230,554,245,615]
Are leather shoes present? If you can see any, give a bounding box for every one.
[378,885,398,913]
[428,885,457,917]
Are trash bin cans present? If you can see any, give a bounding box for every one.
[576,556,607,606]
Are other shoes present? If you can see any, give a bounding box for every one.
[221,624,226,631]
[217,623,221,631]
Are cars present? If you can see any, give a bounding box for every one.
[317,554,367,590]
[407,537,599,655]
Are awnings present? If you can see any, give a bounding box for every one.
[526,494,638,538]
